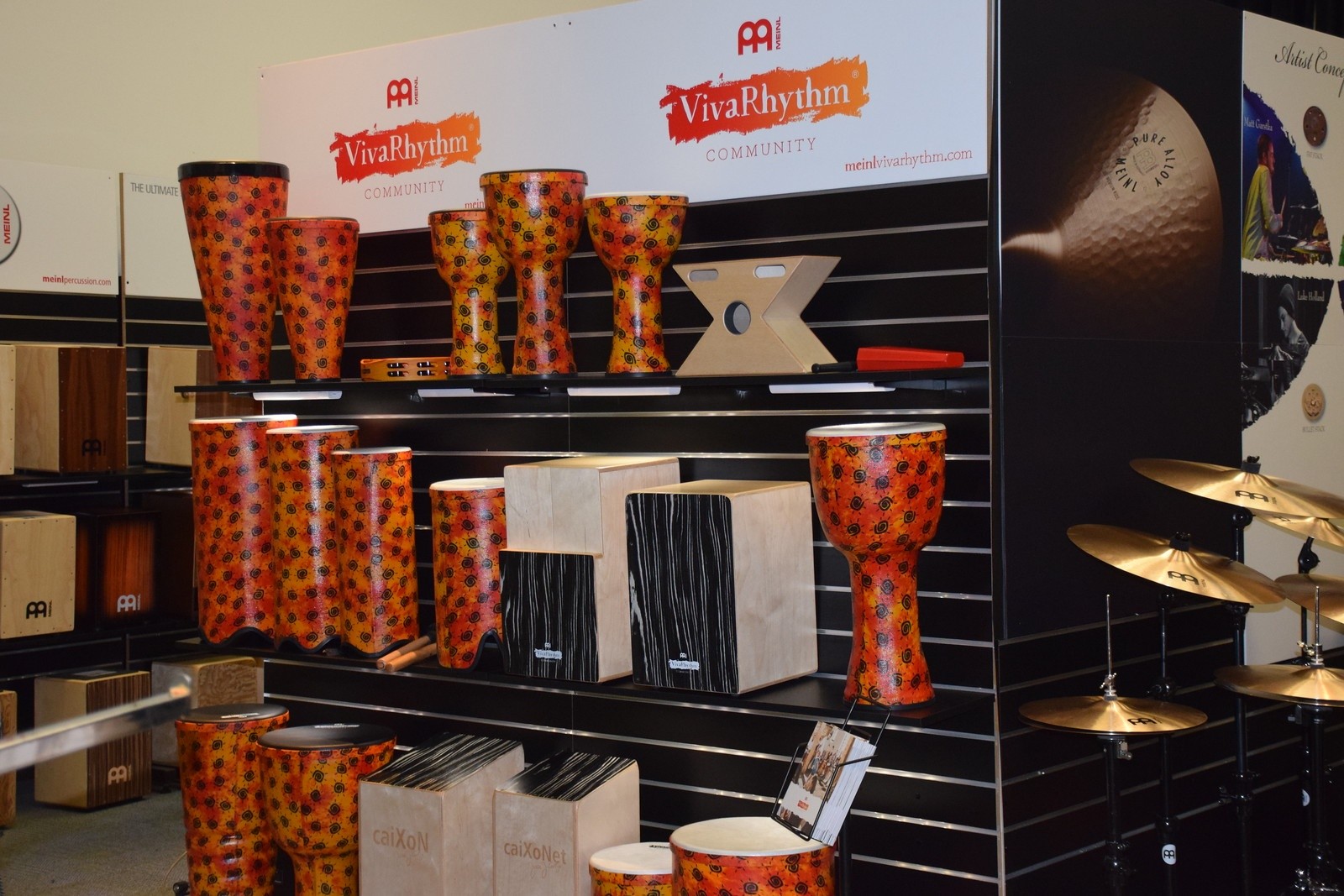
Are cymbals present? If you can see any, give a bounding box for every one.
[1214,665,1344,708]
[1016,696,1208,736]
[1274,574,1344,624]
[1129,455,1344,521]
[1067,524,1287,603]
[1247,508,1344,547]
[360,356,451,381]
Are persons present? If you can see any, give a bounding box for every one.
[1276,283,1310,371]
[1243,133,1284,339]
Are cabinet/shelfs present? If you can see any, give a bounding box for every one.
[0,288,987,896]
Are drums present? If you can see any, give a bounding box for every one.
[804,422,948,712]
[669,816,838,896]
[187,413,420,658]
[177,161,290,383]
[588,841,672,896]
[428,207,512,375]
[256,726,397,896]
[479,169,588,375]
[429,476,508,670]
[174,705,292,896]
[578,193,691,373]
[264,216,360,381]
